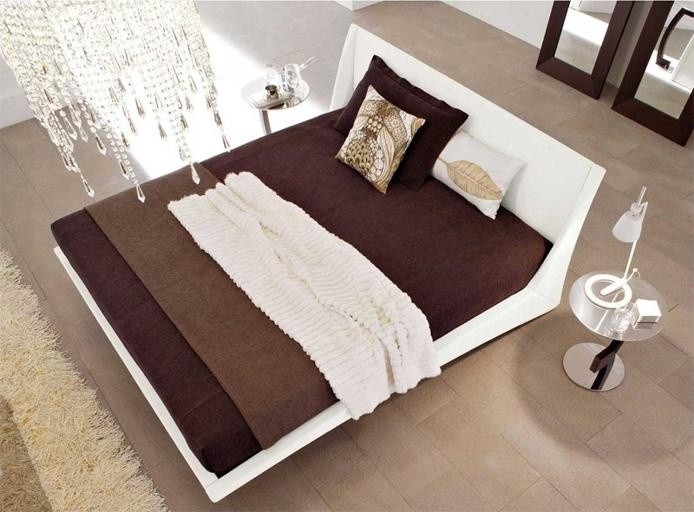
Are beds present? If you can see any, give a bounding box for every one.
[49,16,605,506]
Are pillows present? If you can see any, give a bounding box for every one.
[327,53,469,194]
[334,82,425,197]
[432,127,528,222]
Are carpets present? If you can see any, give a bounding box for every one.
[0,249,172,510]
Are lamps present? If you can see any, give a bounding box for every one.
[1,3,230,205]
[583,180,649,309]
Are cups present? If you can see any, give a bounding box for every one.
[264,85,278,101]
[281,63,301,91]
[610,301,634,334]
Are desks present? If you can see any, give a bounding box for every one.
[562,271,666,392]
[237,79,309,138]
